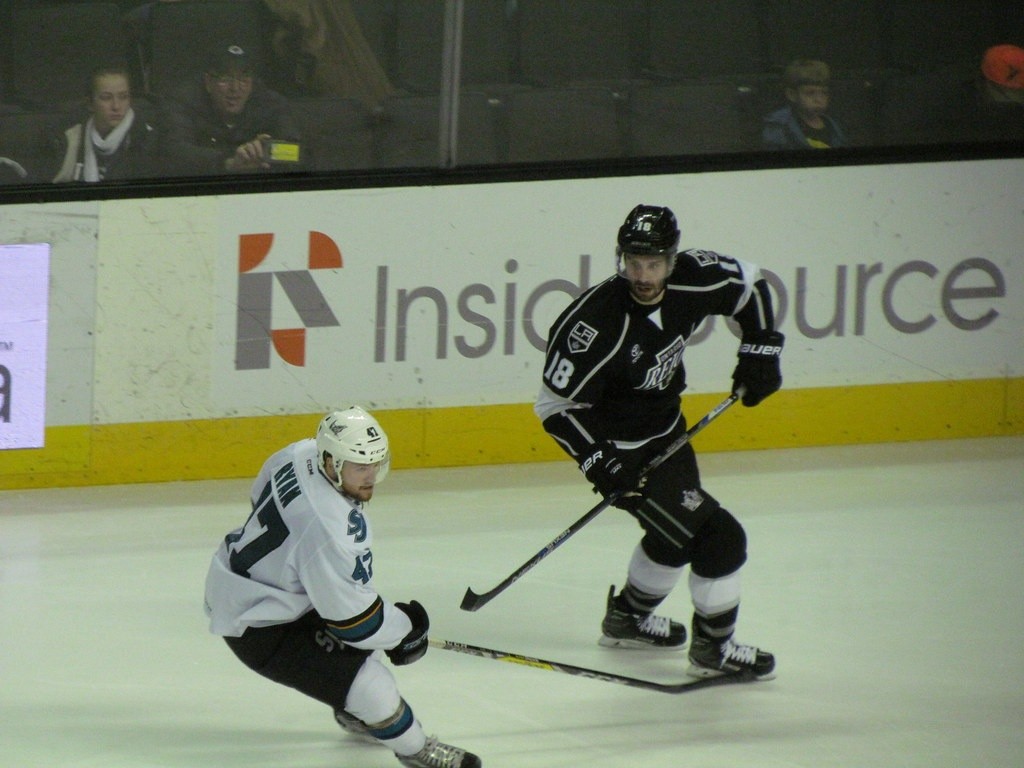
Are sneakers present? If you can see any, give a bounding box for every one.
[687,632,776,682]
[396,736,482,768]
[334,709,380,744]
[598,585,689,651]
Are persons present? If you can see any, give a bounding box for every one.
[532,204,786,681]
[18,68,167,184]
[157,42,317,177]
[753,60,844,151]
[205,406,482,768]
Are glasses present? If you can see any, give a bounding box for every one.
[210,73,248,87]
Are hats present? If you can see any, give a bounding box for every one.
[206,43,251,70]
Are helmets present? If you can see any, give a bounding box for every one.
[315,405,388,472]
[618,204,679,259]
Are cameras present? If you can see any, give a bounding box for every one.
[262,138,305,166]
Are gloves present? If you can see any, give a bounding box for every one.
[576,440,650,510]
[384,600,430,666]
[732,330,785,407]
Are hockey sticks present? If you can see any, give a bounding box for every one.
[459,379,745,614]
[426,635,774,695]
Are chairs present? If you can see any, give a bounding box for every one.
[0,0,1024,179]
